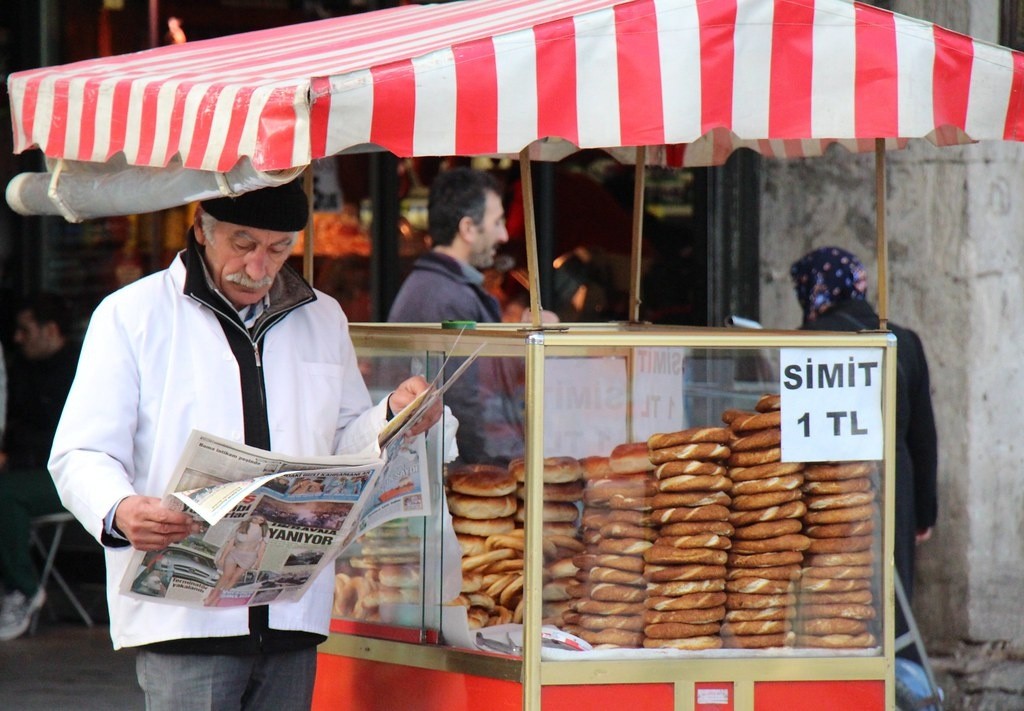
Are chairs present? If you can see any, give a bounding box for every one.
[26,512,94,634]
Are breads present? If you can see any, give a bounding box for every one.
[332,392,883,653]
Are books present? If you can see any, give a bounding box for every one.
[118,322,489,604]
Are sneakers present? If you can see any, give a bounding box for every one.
[0,580,47,639]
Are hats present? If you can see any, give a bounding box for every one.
[198,178,309,232]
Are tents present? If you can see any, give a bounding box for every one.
[10,0,1024,627]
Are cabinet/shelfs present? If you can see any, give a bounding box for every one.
[309,321,897,711]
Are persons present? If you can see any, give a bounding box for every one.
[0,293,80,642]
[382,170,562,469]
[275,510,348,531]
[47,184,443,710]
[787,247,936,660]
[269,474,367,495]
[135,570,163,597]
[204,513,271,607]
